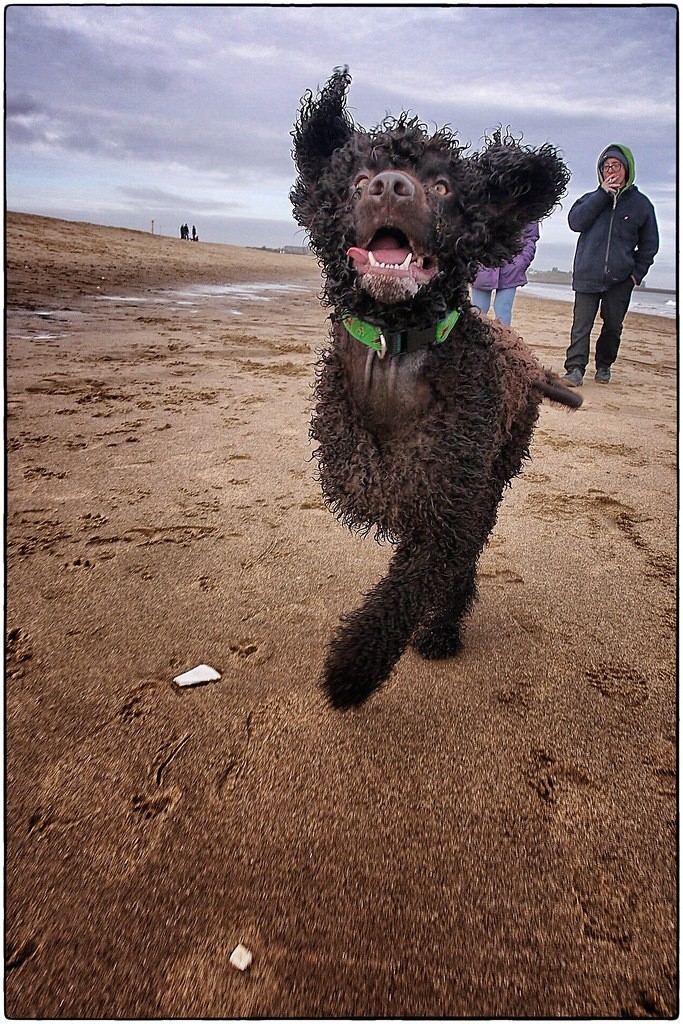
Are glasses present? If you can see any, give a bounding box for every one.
[601,164,624,172]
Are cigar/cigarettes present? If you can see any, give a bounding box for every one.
[610,178,612,183]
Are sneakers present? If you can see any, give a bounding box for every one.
[561,367,585,387]
[595,366,611,384]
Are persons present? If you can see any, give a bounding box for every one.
[559,144,659,388]
[470,218,541,331]
[179,222,199,241]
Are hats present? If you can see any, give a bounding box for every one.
[600,146,628,172]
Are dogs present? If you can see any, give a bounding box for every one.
[291,66,586,712]
